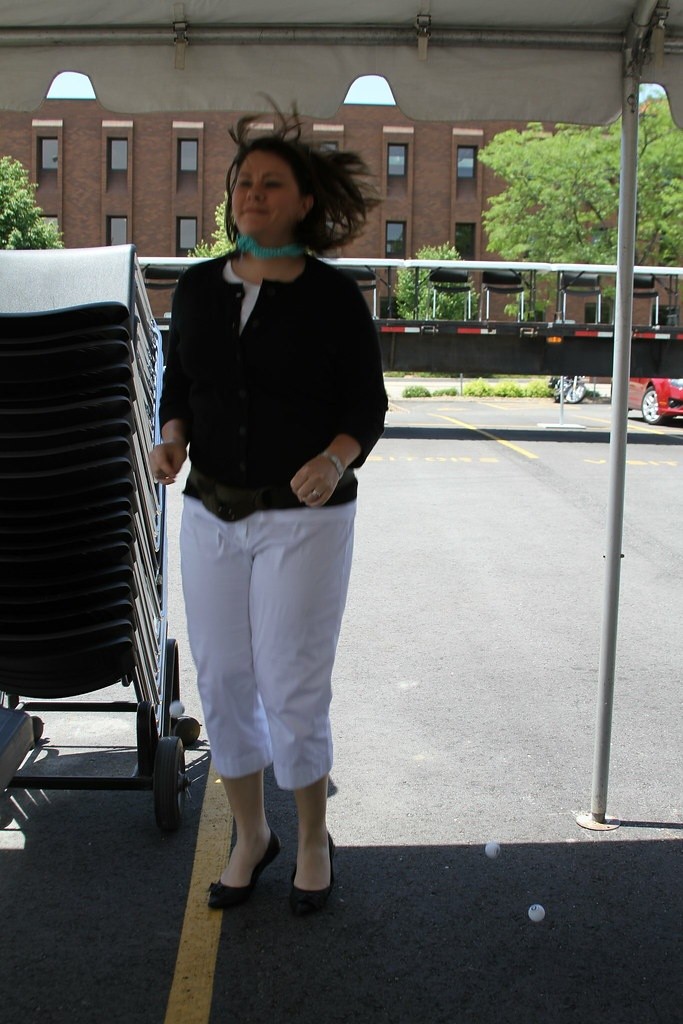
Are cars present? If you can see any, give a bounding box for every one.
[628,377,683,426]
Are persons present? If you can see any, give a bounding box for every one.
[148,92,388,915]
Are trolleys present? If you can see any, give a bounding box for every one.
[0,248,204,831]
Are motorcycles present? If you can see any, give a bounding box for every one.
[549,374,588,403]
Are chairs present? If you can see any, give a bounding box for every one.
[614,275,658,325]
[0,243,167,727]
[478,271,525,321]
[143,266,186,324]
[426,269,472,321]
[338,266,377,318]
[555,271,601,324]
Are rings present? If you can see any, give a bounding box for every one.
[311,489,321,498]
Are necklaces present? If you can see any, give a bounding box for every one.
[236,235,306,261]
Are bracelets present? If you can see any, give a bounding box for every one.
[321,451,344,479]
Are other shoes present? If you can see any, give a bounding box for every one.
[289,832,334,917]
[208,829,281,909]
